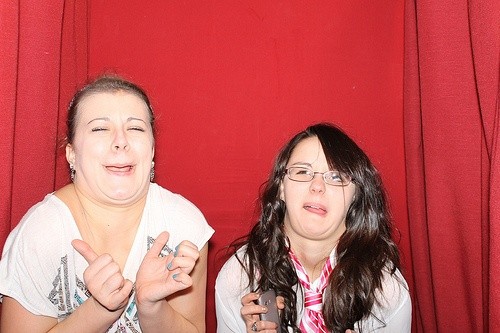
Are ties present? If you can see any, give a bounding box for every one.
[283,245,343,333]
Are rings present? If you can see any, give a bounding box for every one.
[250,321,258,332]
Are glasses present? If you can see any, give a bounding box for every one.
[283,166,356,186]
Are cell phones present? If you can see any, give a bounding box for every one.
[257,288,282,333]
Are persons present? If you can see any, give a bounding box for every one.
[215,122,413,333]
[0,75,215,332]
